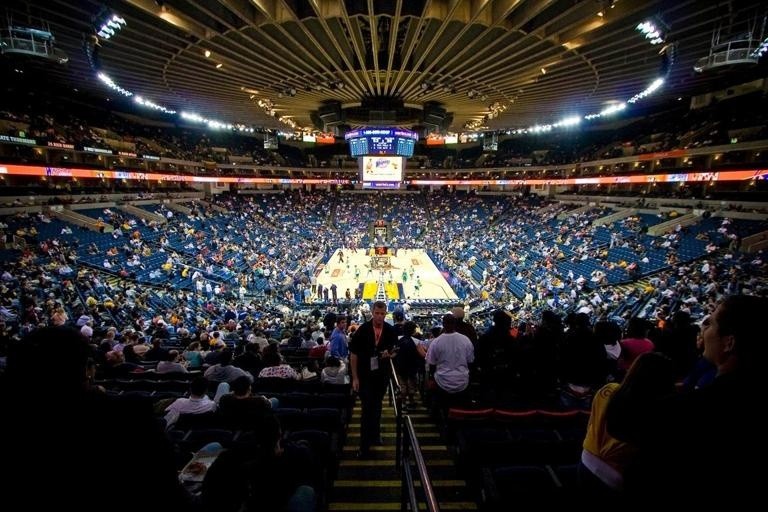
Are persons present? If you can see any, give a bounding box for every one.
[0,110,768,512]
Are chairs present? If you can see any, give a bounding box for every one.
[0,193,768,512]
